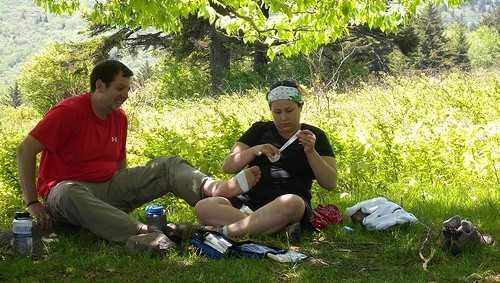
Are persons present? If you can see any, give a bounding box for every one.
[16,60,262,245]
[194,79,338,245]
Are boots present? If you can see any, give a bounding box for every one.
[272,222,302,244]
[177,222,225,242]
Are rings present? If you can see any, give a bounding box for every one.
[263,149,267,152]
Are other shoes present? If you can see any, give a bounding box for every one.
[161,221,181,243]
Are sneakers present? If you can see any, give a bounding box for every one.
[441,214,495,257]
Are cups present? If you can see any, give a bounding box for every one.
[13,212,33,252]
[145,205,166,233]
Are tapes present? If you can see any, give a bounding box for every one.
[269,130,302,163]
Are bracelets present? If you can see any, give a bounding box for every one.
[25,199,39,207]
